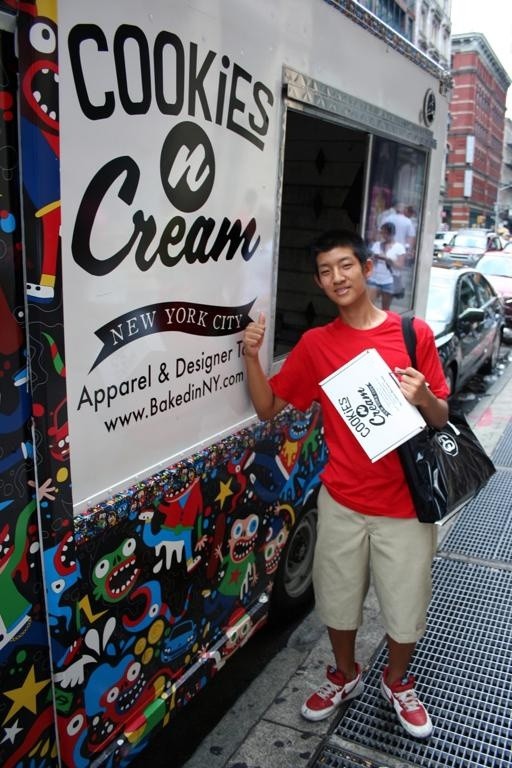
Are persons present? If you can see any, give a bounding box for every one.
[476,207,512,254]
[241,226,451,741]
[375,201,406,240]
[378,205,416,253]
[368,223,407,313]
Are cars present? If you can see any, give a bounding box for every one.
[425,267,504,405]
[433,230,457,261]
[437,229,502,270]
[464,249,512,324]
[498,239,511,253]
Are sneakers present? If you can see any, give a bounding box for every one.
[381,664,433,739]
[301,663,364,721]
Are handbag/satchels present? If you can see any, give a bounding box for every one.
[396,405,497,522]
[393,275,404,298]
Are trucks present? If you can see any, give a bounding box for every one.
[1,1,450,767]
